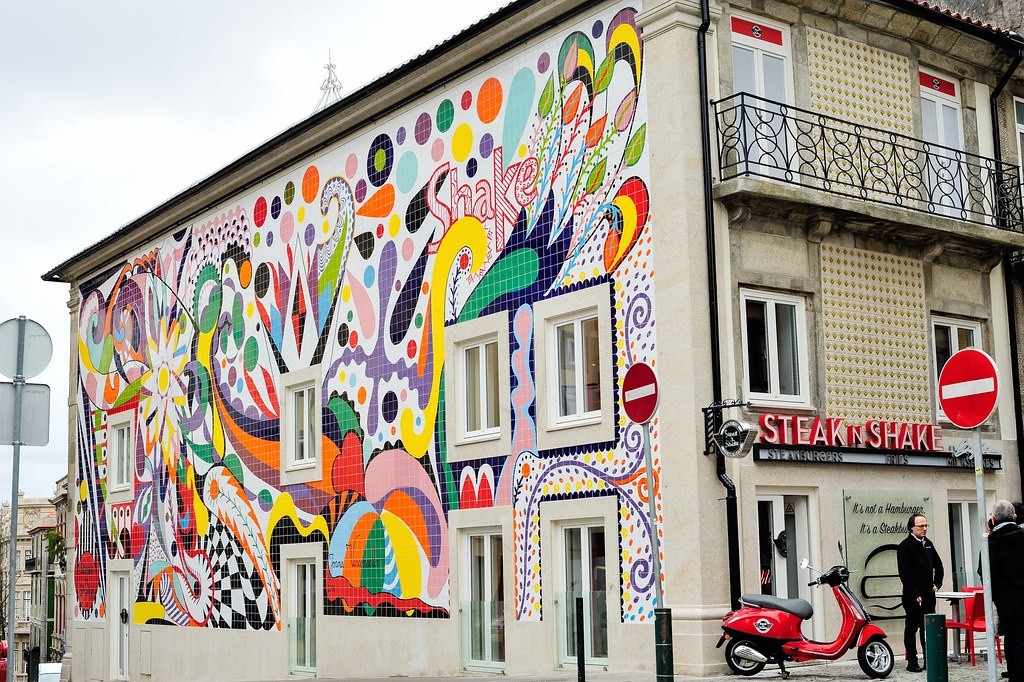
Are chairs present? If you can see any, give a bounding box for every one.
[946,586,1002,664]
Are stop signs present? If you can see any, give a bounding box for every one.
[938,348,999,429]
[621,362,658,425]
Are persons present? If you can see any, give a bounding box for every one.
[977,499,1024,682]
[896,515,967,661]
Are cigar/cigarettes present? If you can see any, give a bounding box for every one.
[919,602,921,606]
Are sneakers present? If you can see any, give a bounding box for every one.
[907,662,922,672]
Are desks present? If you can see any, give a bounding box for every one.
[936,592,988,664]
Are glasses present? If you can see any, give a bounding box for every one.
[914,525,930,529]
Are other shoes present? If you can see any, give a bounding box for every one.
[1001,671,1010,678]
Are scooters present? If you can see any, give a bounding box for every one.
[714,541,894,680]
